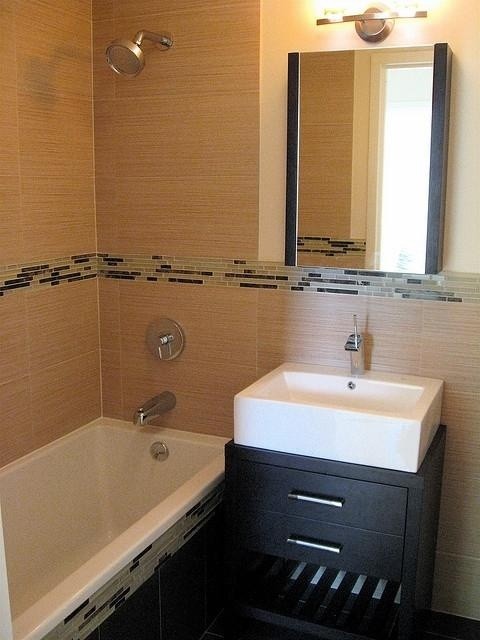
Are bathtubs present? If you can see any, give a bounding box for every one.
[0,415,234,640]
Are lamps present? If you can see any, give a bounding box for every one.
[312,0,428,42]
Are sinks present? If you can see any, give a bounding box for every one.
[233,362,445,472]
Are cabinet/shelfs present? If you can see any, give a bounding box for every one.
[223,423,448,640]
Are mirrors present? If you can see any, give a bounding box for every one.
[285,42,452,275]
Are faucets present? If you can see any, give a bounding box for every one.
[132,390,177,425]
[343,313,366,375]
